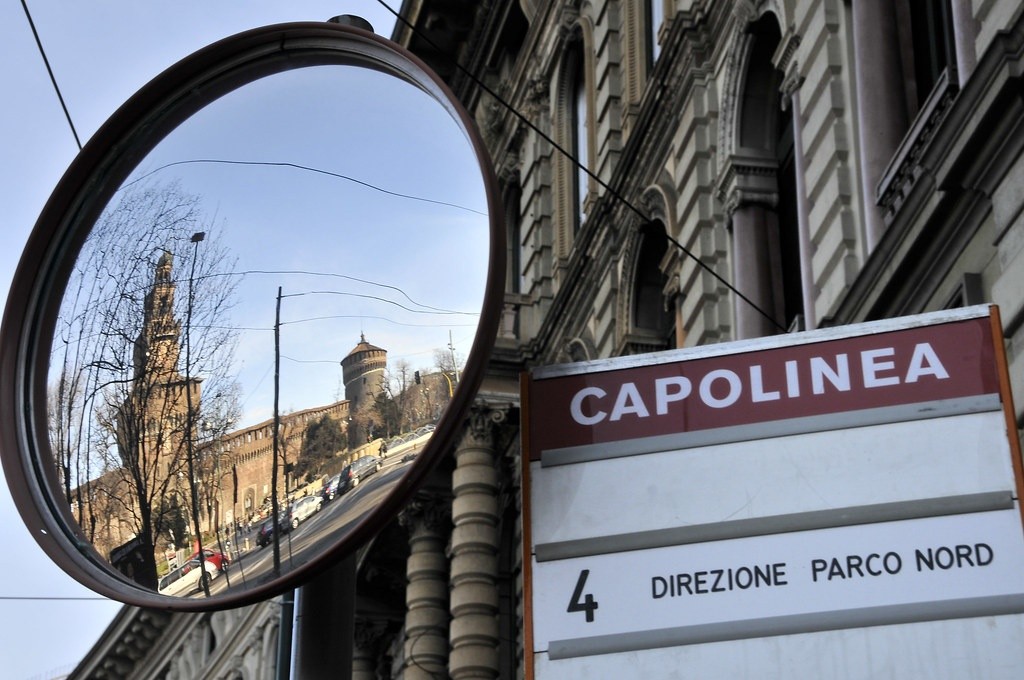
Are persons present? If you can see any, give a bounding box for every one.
[304,487,307,498]
[383,444,388,459]
[222,484,300,537]
[311,486,315,496]
[260,522,268,548]
[378,445,383,460]
[225,523,231,546]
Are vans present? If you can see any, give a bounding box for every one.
[156,559,218,598]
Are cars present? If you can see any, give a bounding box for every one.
[184,549,231,576]
[320,475,343,506]
[284,494,323,528]
[339,453,382,495]
[255,513,291,549]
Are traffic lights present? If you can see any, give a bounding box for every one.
[414,371,422,386]
